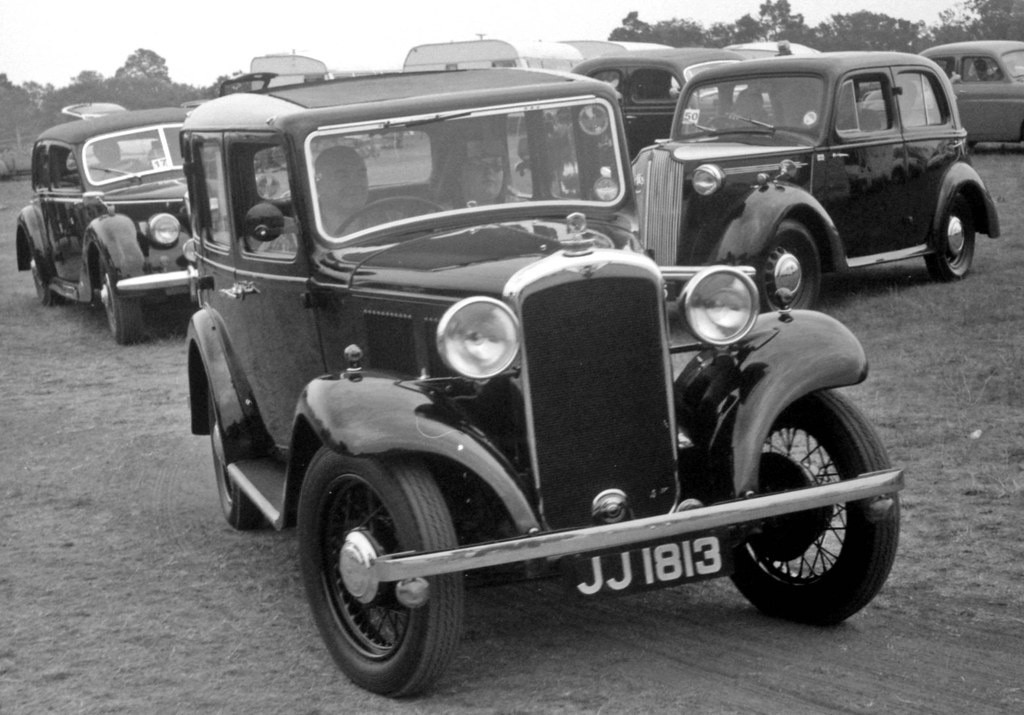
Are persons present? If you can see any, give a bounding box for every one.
[429,141,528,210]
[709,90,770,132]
[258,146,386,253]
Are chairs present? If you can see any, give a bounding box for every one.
[852,109,882,131]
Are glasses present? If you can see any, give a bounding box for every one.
[464,160,504,177]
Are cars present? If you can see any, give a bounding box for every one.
[15,105,222,346]
[914,39,1024,153]
[184,69,905,695]
[626,50,1003,316]
[515,47,771,200]
